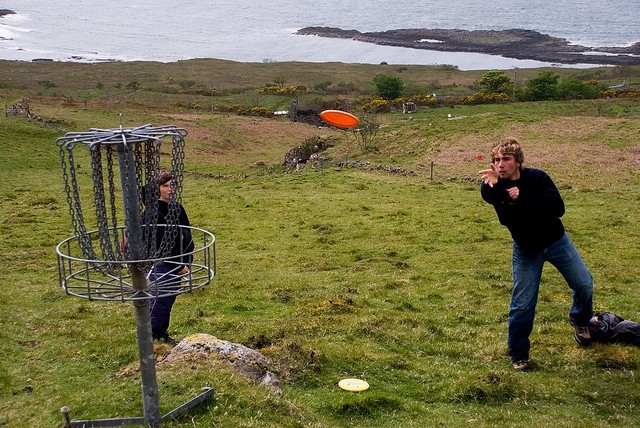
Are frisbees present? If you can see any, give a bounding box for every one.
[339,379,370,391]
[321,110,359,128]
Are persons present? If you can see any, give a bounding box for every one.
[478,134,596,372]
[145,170,195,346]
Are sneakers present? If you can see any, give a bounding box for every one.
[510,355,528,370]
[568,319,591,345]
